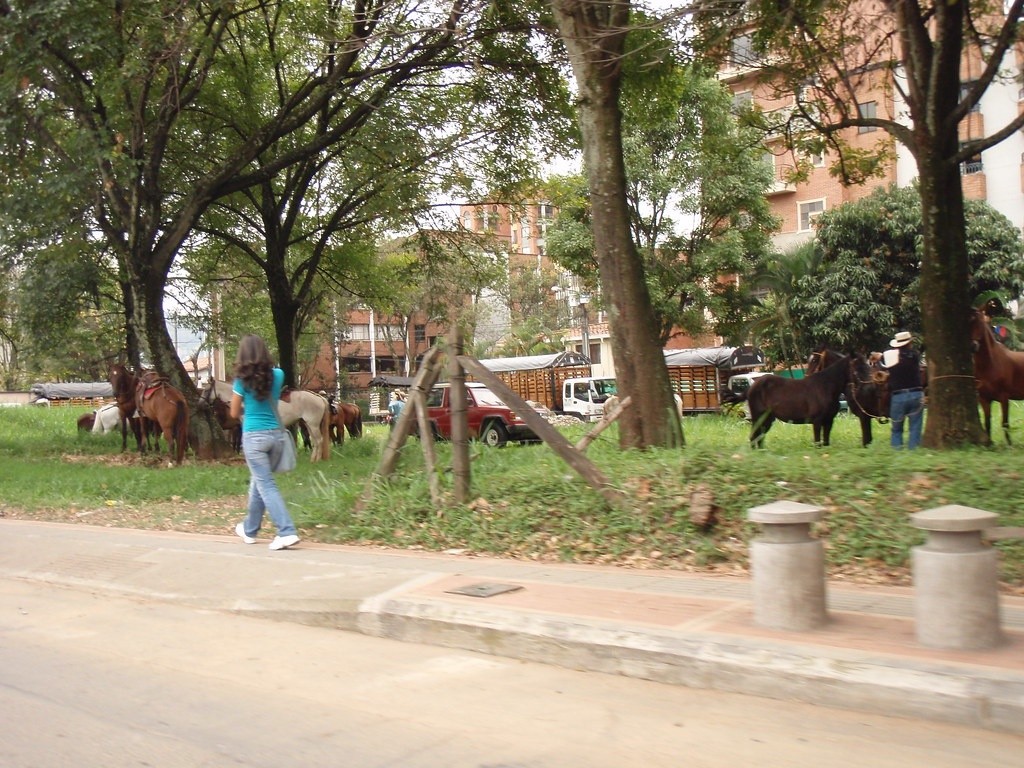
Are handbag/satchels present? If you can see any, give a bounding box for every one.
[274,429,297,471]
[385,414,393,425]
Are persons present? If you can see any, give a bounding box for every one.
[229,336,301,550]
[869,332,924,451]
[388,390,407,430]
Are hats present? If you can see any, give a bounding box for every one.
[394,391,408,402]
[889,331,913,348]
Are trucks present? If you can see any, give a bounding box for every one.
[662,347,776,422]
[460,350,598,425]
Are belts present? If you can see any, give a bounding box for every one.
[893,387,924,395]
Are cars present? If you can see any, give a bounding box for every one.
[409,382,544,447]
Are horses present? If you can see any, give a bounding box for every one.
[717,303,1024,458]
[76,350,363,473]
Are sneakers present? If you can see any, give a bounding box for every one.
[235,523,256,544]
[268,535,301,550]
